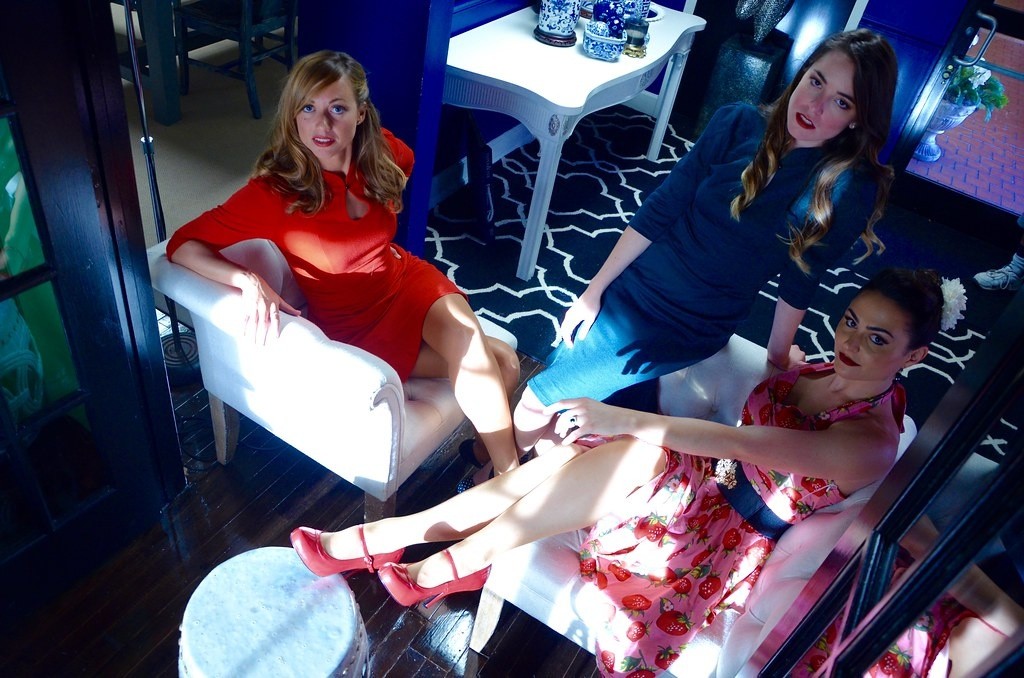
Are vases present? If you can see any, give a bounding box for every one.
[913,99,976,163]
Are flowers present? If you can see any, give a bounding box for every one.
[940,276,968,331]
[943,34,1010,123]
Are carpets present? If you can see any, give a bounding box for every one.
[424,105,1024,464]
[848,201,1016,330]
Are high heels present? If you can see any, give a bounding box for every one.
[289,523,405,578]
[458,438,491,470]
[377,549,492,609]
[458,464,489,493]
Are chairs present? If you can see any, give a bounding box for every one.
[146,238,518,523]
[467,335,917,678]
[173,0,298,120]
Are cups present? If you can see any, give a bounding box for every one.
[583,25,627,63]
[626,19,649,59]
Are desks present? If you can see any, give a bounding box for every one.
[442,2,706,283]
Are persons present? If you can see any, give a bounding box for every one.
[456,28,896,496]
[291,268,969,678]
[973,211,1024,290]
[166,50,520,478]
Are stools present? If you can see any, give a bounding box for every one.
[178,546,371,678]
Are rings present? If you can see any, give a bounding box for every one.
[570,416,577,427]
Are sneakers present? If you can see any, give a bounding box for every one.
[973,267,1019,294]
